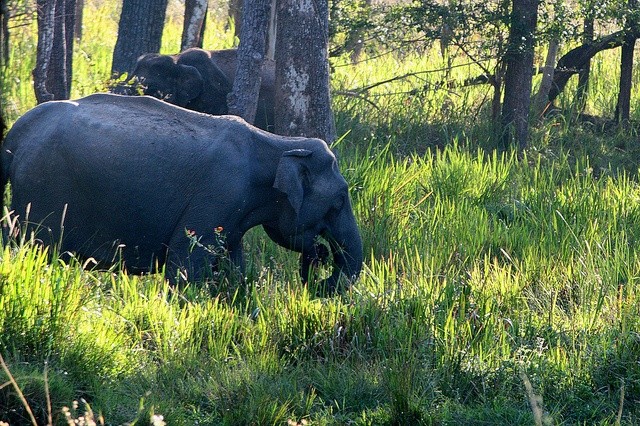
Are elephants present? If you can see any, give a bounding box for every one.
[1,91,364,307]
[119,45,276,134]
